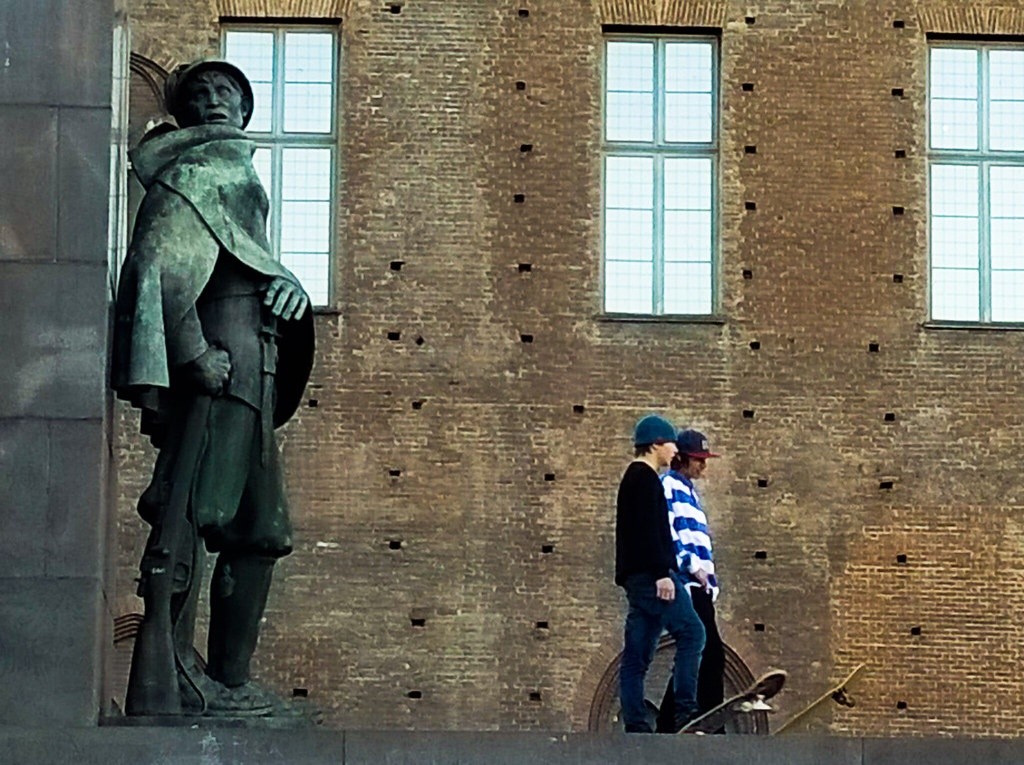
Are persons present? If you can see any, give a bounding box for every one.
[655,429,727,738]
[610,414,707,738]
[114,59,320,715]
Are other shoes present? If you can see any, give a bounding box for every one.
[625,724,657,733]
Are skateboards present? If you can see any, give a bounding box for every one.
[768,662,871,736]
[670,666,790,734]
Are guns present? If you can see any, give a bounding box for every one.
[123,354,217,715]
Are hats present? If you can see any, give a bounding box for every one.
[634,415,677,443]
[678,429,722,458]
